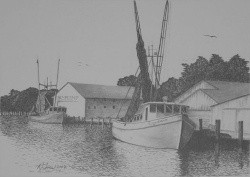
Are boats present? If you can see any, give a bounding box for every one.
[112,102,196,149]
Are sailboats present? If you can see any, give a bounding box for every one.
[28,58,67,123]
